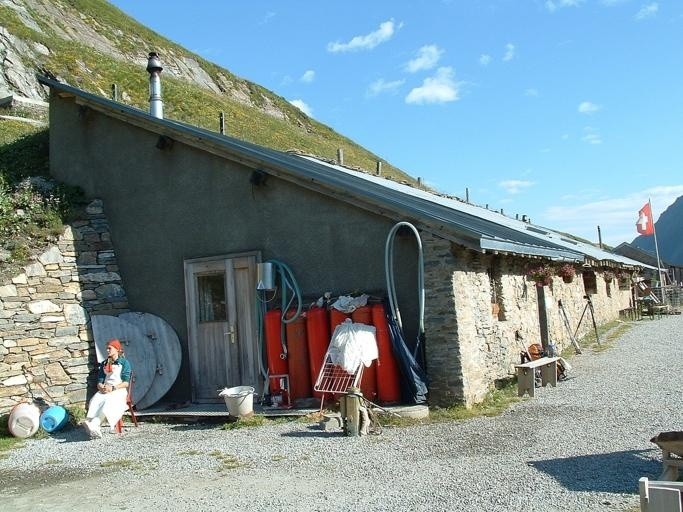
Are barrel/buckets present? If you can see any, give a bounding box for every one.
[220,385,254,417]
[40,406,69,433]
[8,401,40,439]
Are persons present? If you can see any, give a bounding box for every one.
[81,338,131,440]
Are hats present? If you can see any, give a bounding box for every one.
[108,339,121,350]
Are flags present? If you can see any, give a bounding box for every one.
[635,203,654,236]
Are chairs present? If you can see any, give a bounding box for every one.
[117,370,139,433]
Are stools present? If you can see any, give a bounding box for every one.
[653,305,668,320]
[624,307,635,321]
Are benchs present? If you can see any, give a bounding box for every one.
[515,357,562,397]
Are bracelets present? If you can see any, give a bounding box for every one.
[112,386,117,392]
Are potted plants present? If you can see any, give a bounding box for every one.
[602,271,629,283]
[527,262,575,286]
